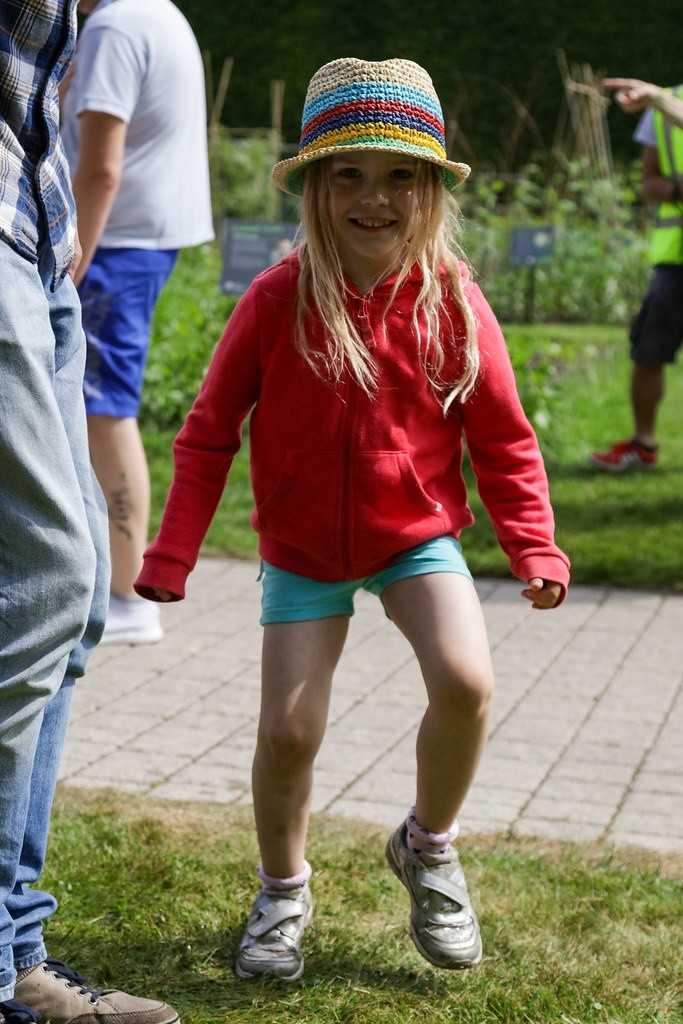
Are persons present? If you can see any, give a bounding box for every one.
[592,78,683,471]
[132,57,572,981]
[0,0,184,1024]
[54,0,215,647]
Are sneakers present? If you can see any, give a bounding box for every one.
[100,595,164,645]
[234,866,313,981]
[384,815,486,971]
[0,964,179,1024]
[594,441,660,475]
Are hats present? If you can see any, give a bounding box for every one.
[270,55,470,197]
